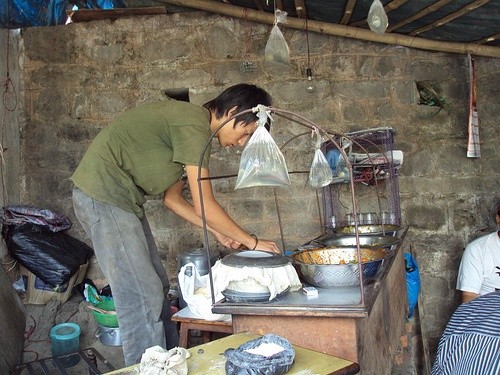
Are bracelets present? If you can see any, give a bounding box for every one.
[250,234,258,251]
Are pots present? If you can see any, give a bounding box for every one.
[92,325,124,347]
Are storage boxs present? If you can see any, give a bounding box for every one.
[19,262,89,305]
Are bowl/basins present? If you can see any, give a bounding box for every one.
[333,223,405,238]
[292,237,405,289]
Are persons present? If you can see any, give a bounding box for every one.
[455,198,500,303]
[69,83,281,368]
[431,289,500,375]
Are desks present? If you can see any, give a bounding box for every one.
[104,331,361,375]
[212,223,410,375]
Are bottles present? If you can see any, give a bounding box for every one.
[329,212,397,228]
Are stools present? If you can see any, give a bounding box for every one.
[171,307,233,349]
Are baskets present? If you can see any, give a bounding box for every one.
[93,300,119,328]
